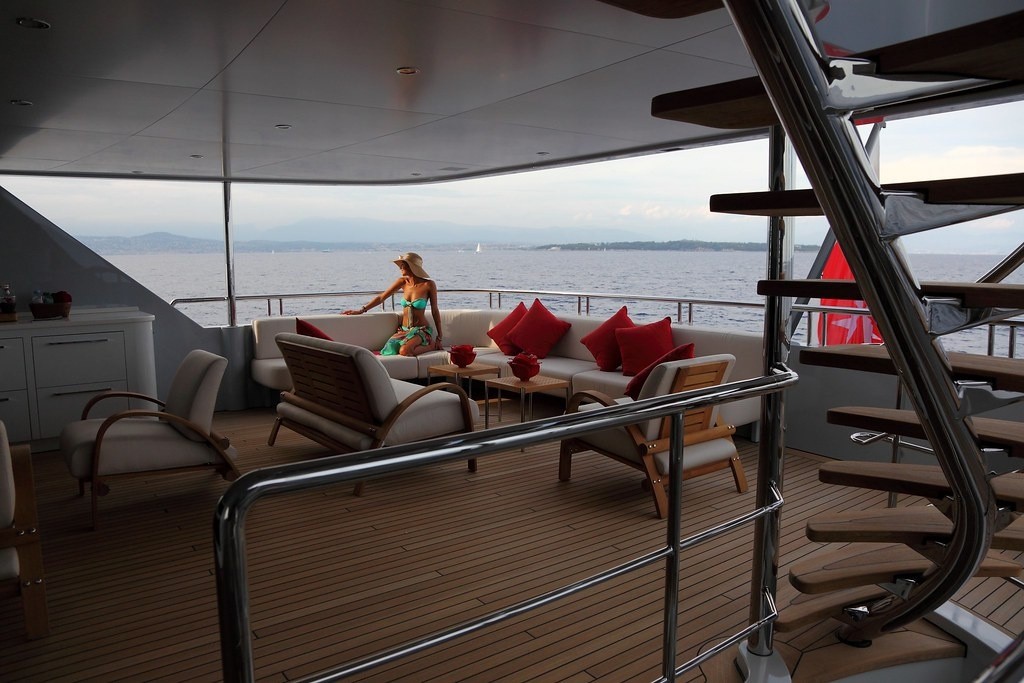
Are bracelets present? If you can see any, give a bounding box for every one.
[437,336,442,340]
[363,306,367,312]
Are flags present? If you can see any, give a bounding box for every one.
[817,134,884,348]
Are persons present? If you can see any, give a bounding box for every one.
[343,252,443,355]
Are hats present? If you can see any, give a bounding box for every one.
[393,253,430,279]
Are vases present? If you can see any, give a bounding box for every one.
[448,345,477,368]
[508,353,540,382]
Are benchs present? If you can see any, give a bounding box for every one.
[267,331,480,497]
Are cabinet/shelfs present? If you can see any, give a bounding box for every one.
[0,311,159,453]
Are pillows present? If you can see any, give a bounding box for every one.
[580,305,694,399]
[296,317,334,341]
[488,302,573,355]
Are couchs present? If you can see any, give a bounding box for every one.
[251,309,763,442]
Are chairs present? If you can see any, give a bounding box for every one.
[59,348,241,526]
[0,420,50,640]
[559,353,749,517]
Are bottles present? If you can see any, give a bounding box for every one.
[32,290,53,305]
[3,284,11,297]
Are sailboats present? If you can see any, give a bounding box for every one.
[473,240,482,255]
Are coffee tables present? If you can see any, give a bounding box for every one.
[427,364,501,422]
[485,375,571,452]
